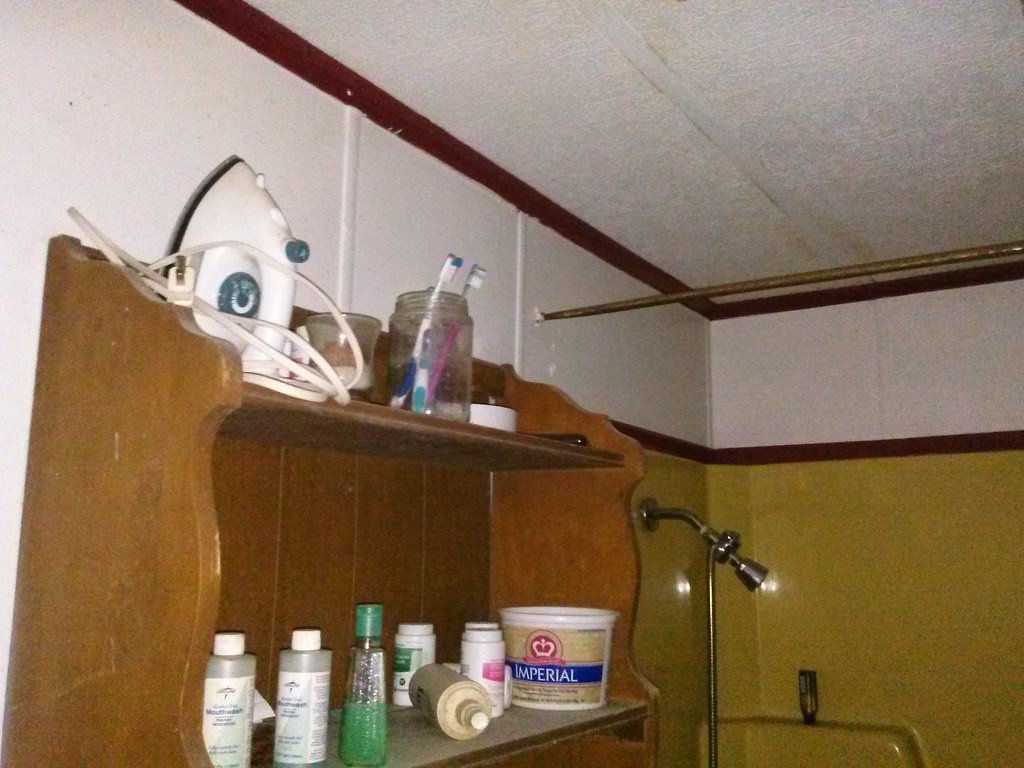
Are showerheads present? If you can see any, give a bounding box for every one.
[638,493,769,592]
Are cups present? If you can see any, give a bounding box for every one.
[304,311,384,399]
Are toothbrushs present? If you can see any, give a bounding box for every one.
[409,360,428,414]
[421,262,486,408]
[390,250,464,407]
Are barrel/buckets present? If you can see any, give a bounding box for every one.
[498,605,621,711]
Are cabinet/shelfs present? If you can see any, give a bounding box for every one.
[0,237,662,768]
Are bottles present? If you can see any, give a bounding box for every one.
[461,622,497,650]
[393,622,435,707]
[407,662,492,740]
[388,290,474,423]
[442,660,513,710]
[339,602,388,768]
[460,631,506,719]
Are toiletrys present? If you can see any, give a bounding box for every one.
[339,601,393,768]
